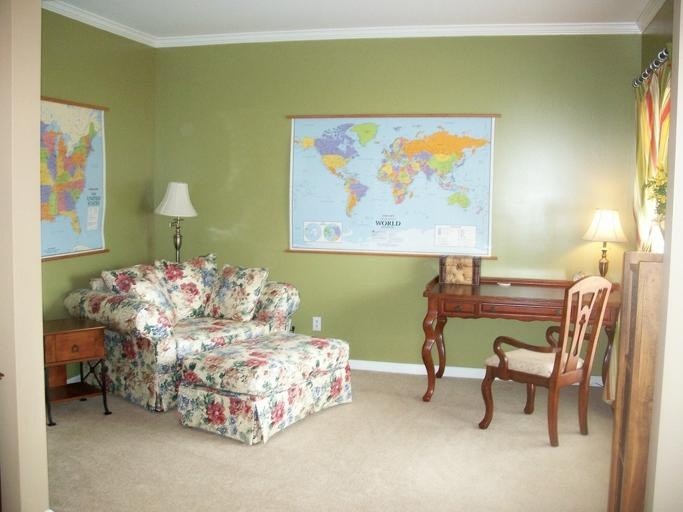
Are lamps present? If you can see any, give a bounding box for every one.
[583,210,628,278]
[153,181,197,261]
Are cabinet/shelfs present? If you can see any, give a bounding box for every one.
[607,248,665,512]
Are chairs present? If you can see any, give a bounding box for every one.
[478,274,612,445]
[61,253,298,415]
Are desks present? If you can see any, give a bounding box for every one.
[420,275,620,402]
[42,316,112,427]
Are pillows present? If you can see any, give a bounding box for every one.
[153,253,217,319]
[205,264,269,320]
[98,265,178,327]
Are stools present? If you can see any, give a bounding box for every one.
[175,331,352,443]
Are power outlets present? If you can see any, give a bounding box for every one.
[312,316,321,332]
